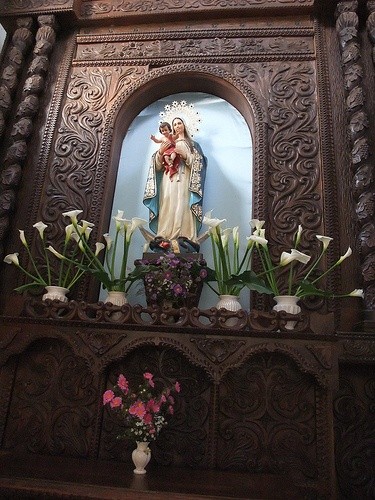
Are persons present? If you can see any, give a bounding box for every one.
[143,118,207,240]
[150,122,180,167]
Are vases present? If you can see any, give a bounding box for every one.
[273,295,302,331]
[215,294,242,325]
[131,440,152,474]
[105,291,128,317]
[41,286,69,302]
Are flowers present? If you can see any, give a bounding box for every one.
[3,209,365,300]
[102,371,182,444]
